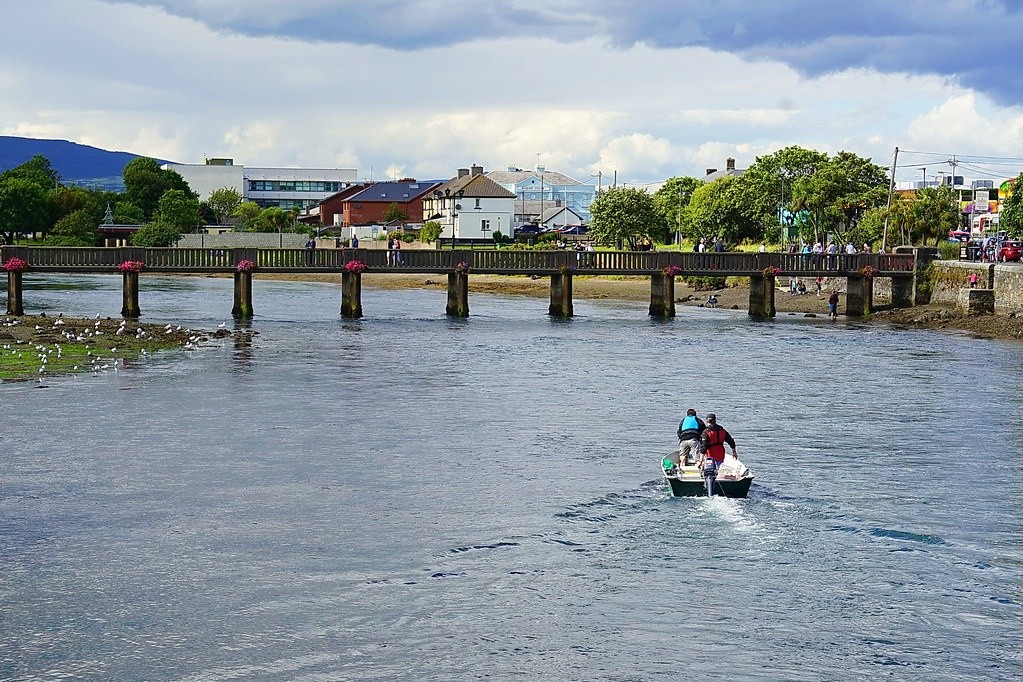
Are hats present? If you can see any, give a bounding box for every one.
[706,414,715,421]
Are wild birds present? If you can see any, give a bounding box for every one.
[3,313,200,383]
[218,322,226,327]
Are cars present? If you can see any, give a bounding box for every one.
[514,224,590,235]
[948,230,1023,262]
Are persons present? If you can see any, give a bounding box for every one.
[676,409,707,466]
[695,413,737,476]
[789,276,823,296]
[964,272,978,290]
[704,296,718,308]
[827,290,839,316]
[977,233,996,263]
[302,233,888,273]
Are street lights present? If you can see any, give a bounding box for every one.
[444,187,465,249]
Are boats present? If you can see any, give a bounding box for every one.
[660,448,755,499]
